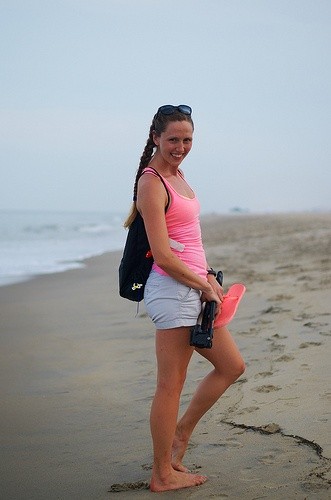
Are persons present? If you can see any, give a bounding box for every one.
[118,103,246,493]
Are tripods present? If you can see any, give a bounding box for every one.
[190,271,223,349]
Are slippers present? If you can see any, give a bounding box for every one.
[213,284,246,329]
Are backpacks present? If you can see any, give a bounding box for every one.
[119,166,171,302]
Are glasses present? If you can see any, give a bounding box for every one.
[155,105,192,119]
